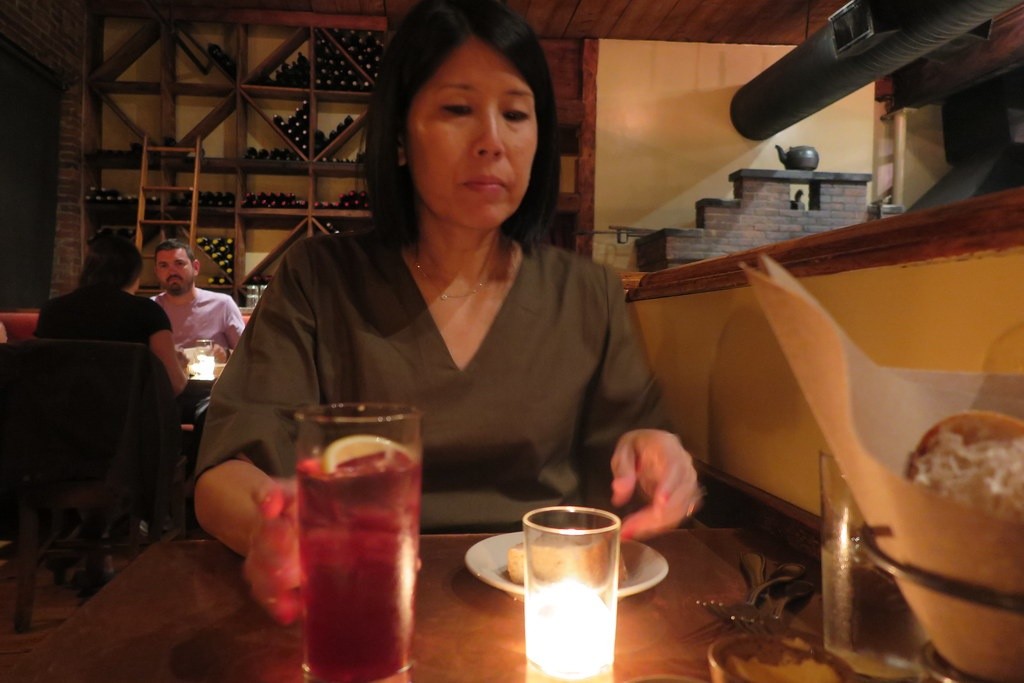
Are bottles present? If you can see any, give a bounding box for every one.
[806,431,937,683]
[85,27,383,285]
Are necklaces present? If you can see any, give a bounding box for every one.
[412,243,511,301]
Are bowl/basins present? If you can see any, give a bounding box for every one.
[707,634,854,683]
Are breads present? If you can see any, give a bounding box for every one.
[907,411,1023,481]
[507,529,627,585]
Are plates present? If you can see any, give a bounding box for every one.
[464,531,669,602]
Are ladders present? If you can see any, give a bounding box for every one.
[134,134,204,296]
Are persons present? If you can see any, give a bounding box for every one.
[36,237,187,583]
[192,0,707,623]
[148,241,246,535]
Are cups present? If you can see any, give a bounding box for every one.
[196,339,213,356]
[521,506,621,683]
[292,401,424,683]
[245,283,268,306]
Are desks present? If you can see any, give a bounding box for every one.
[2,527,921,683]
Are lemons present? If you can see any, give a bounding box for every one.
[320,435,410,474]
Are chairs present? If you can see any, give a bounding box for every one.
[0,339,185,635]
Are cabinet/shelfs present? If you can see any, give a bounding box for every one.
[82,13,393,316]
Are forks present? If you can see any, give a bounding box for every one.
[695,548,815,637]
